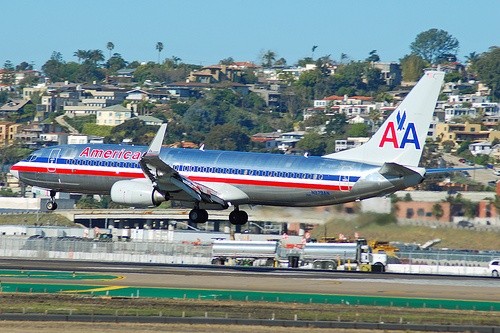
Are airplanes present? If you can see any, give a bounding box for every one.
[9,71,445,225]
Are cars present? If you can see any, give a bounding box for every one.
[489,260,500,277]
